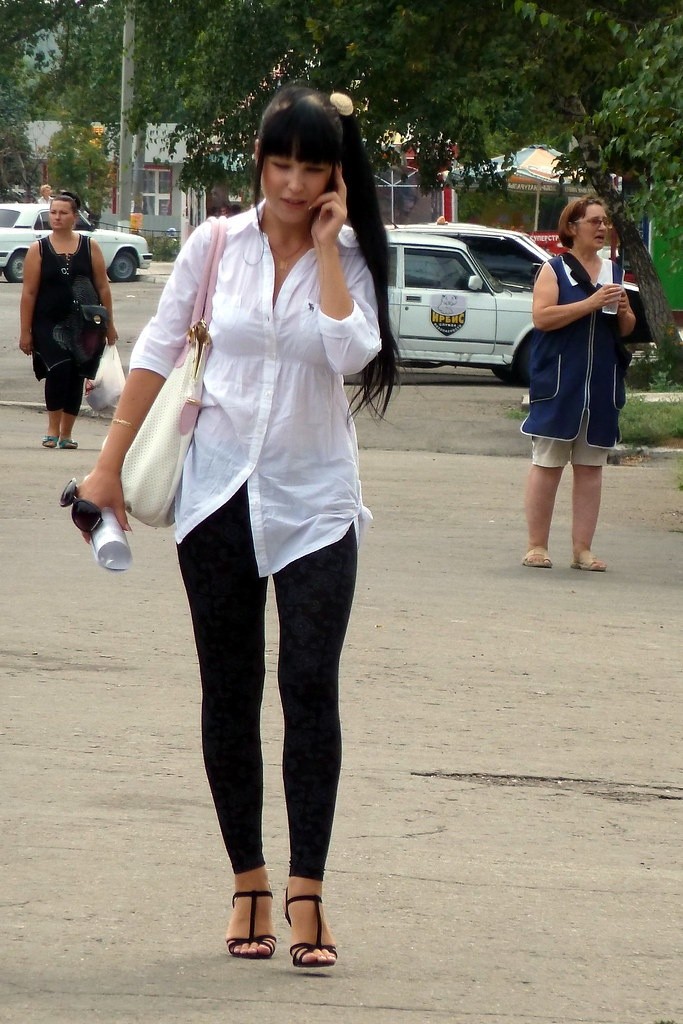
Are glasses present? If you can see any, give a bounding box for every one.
[59,477,103,533]
[571,216,614,230]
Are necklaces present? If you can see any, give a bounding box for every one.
[270,234,309,270]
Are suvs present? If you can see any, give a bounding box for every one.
[384,231,534,388]
[385,223,647,339]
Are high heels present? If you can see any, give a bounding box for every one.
[284,887,338,968]
[226,890,276,958]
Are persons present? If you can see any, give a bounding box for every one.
[77,86,397,967]
[521,196,636,572]
[38,185,54,204]
[19,192,119,449]
[207,204,241,218]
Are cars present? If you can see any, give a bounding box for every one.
[0,203,154,283]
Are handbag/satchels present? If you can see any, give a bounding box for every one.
[98,215,228,529]
[79,303,108,333]
[85,343,125,412]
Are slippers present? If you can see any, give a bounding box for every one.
[571,550,607,571]
[59,438,77,449]
[521,546,553,569]
[41,434,59,448]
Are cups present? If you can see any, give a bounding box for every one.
[602,283,621,314]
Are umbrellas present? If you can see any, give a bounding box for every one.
[440,143,622,232]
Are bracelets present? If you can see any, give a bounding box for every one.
[112,419,139,433]
